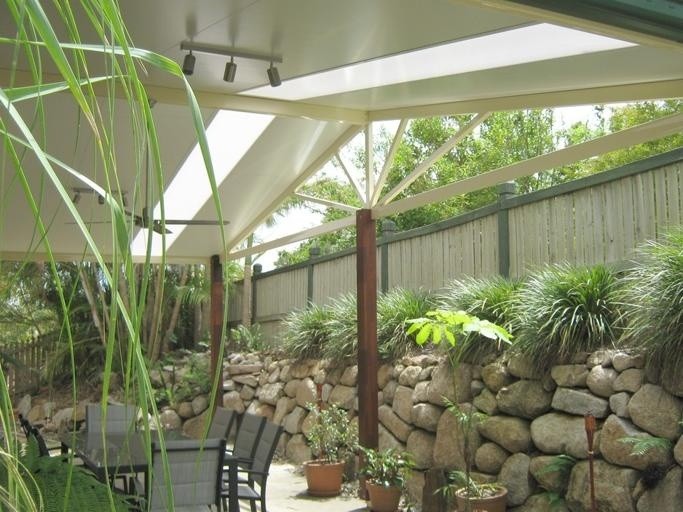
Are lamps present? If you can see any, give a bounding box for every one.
[73,188,128,207]
[181,42,283,87]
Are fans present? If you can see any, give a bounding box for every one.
[64,99,231,235]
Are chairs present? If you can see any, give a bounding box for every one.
[18,404,284,512]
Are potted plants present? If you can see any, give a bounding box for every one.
[356,445,416,512]
[302,401,347,496]
[434,471,509,512]
